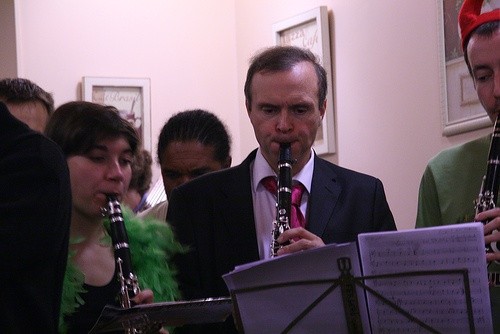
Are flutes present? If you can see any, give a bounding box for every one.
[270,142,292,258]
[101,193,144,334]
[473,109,500,288]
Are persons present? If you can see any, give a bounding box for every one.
[414,0,500,334]
[0,78,53,135]
[166,47,397,334]
[134,110,231,222]
[0,101,73,334]
[43,101,189,334]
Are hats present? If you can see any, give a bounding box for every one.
[456,0,500,52]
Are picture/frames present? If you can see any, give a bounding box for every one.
[435,0,494,137]
[272,6,336,157]
[81,76,152,158]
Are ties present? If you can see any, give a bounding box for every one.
[261,176,305,242]
